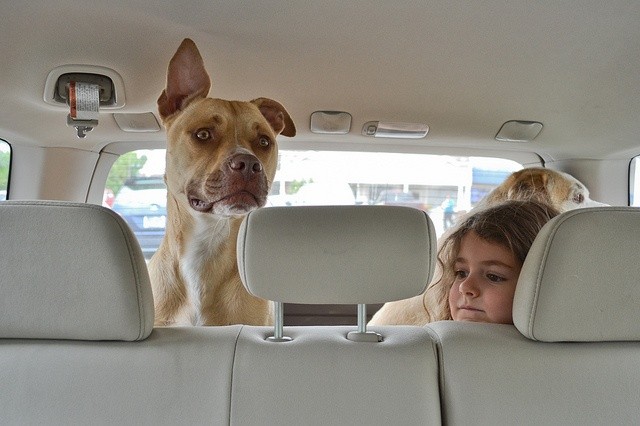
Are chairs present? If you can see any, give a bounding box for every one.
[425,205,639,426]
[228,204,443,426]
[2,202,244,421]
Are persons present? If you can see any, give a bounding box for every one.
[419,193,566,322]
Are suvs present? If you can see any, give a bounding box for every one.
[109,174,169,258]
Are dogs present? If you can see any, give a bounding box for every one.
[367,166,611,327]
[146,35,296,328]
